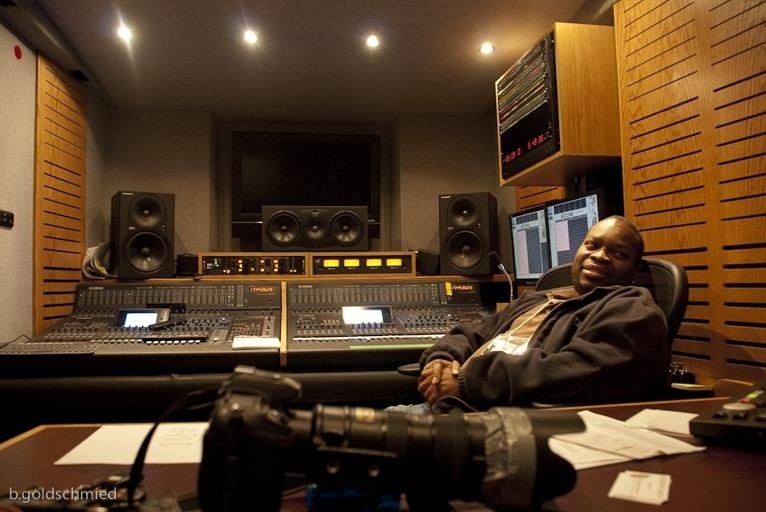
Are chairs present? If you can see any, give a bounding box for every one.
[534,259,687,390]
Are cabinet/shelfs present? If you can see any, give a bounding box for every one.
[496,22,619,189]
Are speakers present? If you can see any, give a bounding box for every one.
[437,192,500,277]
[260,205,369,252]
[111,189,175,279]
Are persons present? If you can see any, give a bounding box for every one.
[382,214,670,417]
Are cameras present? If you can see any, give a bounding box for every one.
[191,364,586,512]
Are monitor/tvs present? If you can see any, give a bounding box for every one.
[115,308,171,328]
[508,204,552,284]
[544,189,605,273]
[341,303,393,325]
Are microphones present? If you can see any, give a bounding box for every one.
[488,251,513,305]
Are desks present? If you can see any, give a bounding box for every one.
[1,395,764,512]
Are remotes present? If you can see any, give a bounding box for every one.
[150,316,186,331]
